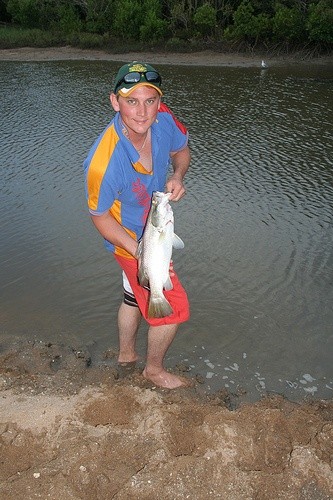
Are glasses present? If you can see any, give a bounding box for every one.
[114,71,162,95]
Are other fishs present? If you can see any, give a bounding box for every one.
[137,190,185,316]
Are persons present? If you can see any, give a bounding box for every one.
[83,62,190,389]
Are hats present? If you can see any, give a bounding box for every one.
[115,62,162,95]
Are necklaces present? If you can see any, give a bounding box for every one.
[138,128,150,153]
